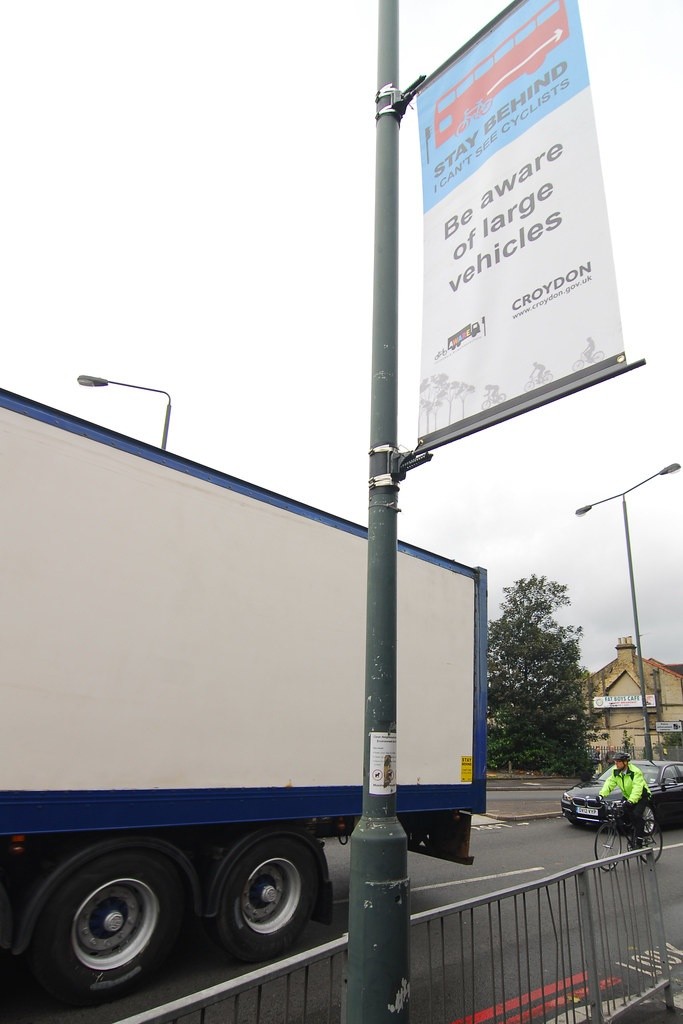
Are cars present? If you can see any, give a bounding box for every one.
[560,760,683,836]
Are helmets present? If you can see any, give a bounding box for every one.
[612,752,631,761]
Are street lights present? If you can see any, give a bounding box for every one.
[78,374,171,454]
[573,463,680,762]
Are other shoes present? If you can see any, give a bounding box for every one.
[635,837,643,848]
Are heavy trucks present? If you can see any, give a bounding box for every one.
[0,383,486,1010]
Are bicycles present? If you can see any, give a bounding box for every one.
[594,799,661,872]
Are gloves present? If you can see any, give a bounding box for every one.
[622,800,632,804]
[594,795,604,805]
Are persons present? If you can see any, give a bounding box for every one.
[596,745,632,769]
[596,751,652,850]
